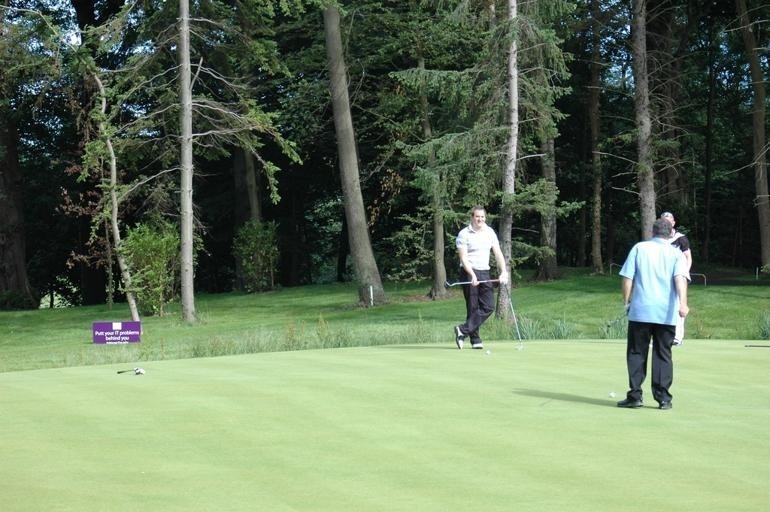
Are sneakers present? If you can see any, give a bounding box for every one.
[472,343,483,349]
[672,337,682,345]
[454,326,465,350]
[618,398,642,407]
[660,402,672,409]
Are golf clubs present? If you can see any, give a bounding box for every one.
[117,368,145,375]
[504,284,522,350]
[444,280,500,290]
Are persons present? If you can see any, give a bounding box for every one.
[617,216,690,410]
[660,211,694,348]
[452,204,508,349]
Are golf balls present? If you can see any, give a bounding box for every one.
[609,392,615,398]
[486,349,490,354]
